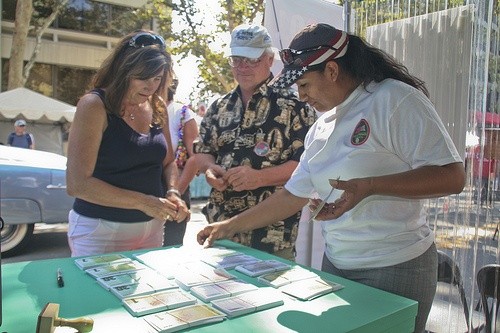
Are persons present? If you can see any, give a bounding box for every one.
[159,68,199,248]
[7,119,34,150]
[191,22,318,262]
[196,22,467,333]
[468,133,494,205]
[66,29,191,257]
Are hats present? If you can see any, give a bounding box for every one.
[14,120,28,126]
[267,23,350,89]
[222,23,273,59]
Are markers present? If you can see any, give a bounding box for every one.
[57,268,64,287]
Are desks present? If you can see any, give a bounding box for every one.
[0,239,418,333]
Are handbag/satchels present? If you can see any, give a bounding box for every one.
[173,104,201,176]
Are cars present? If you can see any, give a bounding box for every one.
[0,144,75,257]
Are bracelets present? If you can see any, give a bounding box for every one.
[166,188,181,197]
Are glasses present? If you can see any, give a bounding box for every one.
[278,44,339,66]
[121,32,167,55]
[227,57,268,67]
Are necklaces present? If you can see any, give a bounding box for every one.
[126,104,138,119]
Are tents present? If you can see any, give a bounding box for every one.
[0,87,78,155]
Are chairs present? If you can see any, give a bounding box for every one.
[436,248,500,332]
